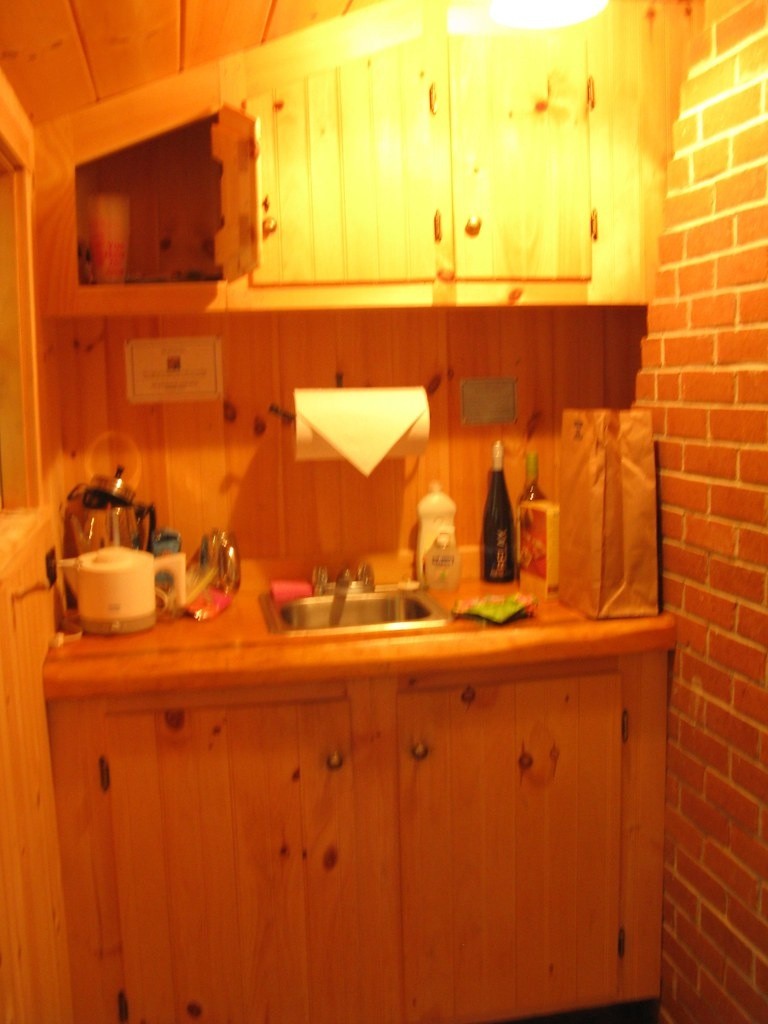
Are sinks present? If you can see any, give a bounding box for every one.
[257,580,452,639]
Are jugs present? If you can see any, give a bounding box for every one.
[66,505,157,558]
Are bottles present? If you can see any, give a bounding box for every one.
[482,440,515,580]
[516,452,547,583]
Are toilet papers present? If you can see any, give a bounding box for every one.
[290,385,434,479]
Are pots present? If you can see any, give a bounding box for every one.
[52,544,188,637]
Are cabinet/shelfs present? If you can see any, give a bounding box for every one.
[33,1,648,317]
[41,620,679,1024]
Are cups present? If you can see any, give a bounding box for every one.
[89,193,130,284]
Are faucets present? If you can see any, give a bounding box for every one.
[335,565,354,594]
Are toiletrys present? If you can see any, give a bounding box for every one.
[412,476,463,587]
